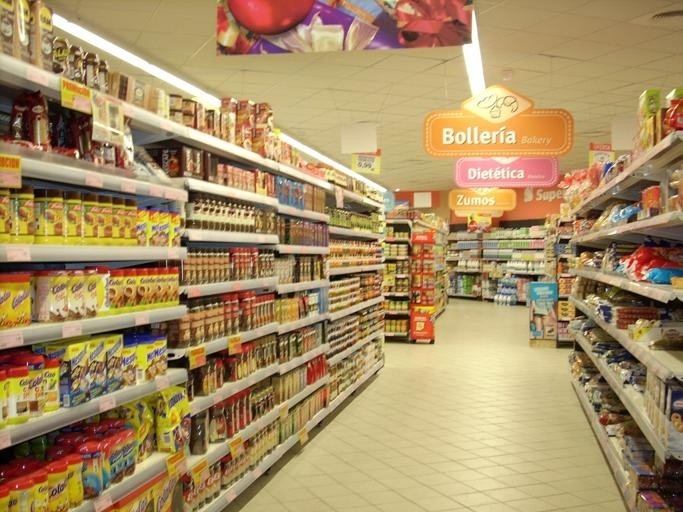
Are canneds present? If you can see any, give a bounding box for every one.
[185,192,277,512]
[0,182,183,249]
[0,351,61,430]
[352,273,377,301]
[0,265,182,330]
[0,415,139,511]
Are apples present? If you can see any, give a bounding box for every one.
[228,0,315,33]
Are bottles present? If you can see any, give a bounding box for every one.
[150,289,281,348]
[175,380,278,455]
[0,349,62,429]
[177,243,280,285]
[0,416,138,512]
[185,421,280,510]
[173,335,279,398]
[180,194,280,234]
